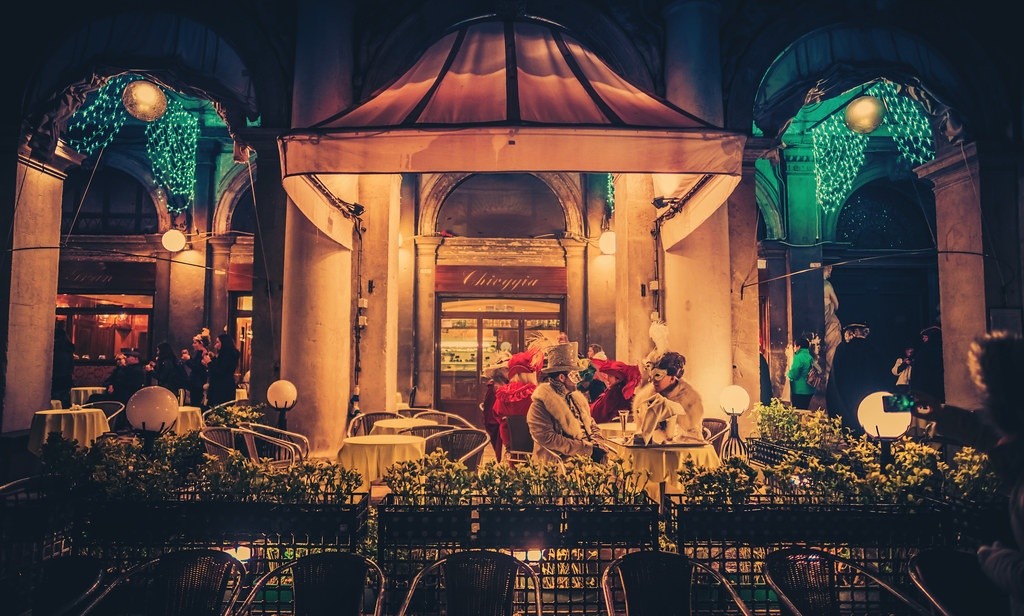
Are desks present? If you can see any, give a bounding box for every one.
[337,434,426,492]
[176,405,208,435]
[602,436,721,512]
[70,387,105,404]
[368,418,438,434]
[595,422,636,440]
[28,408,112,455]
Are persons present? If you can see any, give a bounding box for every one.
[825,316,871,438]
[891,326,944,404]
[51,322,76,410]
[819,266,841,385]
[84,327,242,430]
[908,330,1024,616]
[760,352,773,408]
[787,338,814,409]
[526,337,617,467]
[631,349,721,490]
[482,329,642,463]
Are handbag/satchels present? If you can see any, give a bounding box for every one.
[806,356,826,393]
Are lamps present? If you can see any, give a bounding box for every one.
[122,79,167,122]
[267,380,298,460]
[159,229,186,253]
[599,229,615,255]
[720,384,750,461]
[843,95,884,134]
[858,391,912,475]
[124,386,179,456]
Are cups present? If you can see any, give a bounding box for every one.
[618,409,630,441]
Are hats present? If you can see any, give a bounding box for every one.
[483,350,513,378]
[541,342,591,373]
[507,349,544,379]
[123,352,141,357]
[599,360,642,400]
[841,304,868,330]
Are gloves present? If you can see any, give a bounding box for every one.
[590,444,608,464]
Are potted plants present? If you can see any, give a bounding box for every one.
[6,397,1003,547]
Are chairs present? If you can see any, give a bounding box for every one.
[0,387,541,616]
[530,416,1024,616]
[83,400,124,431]
